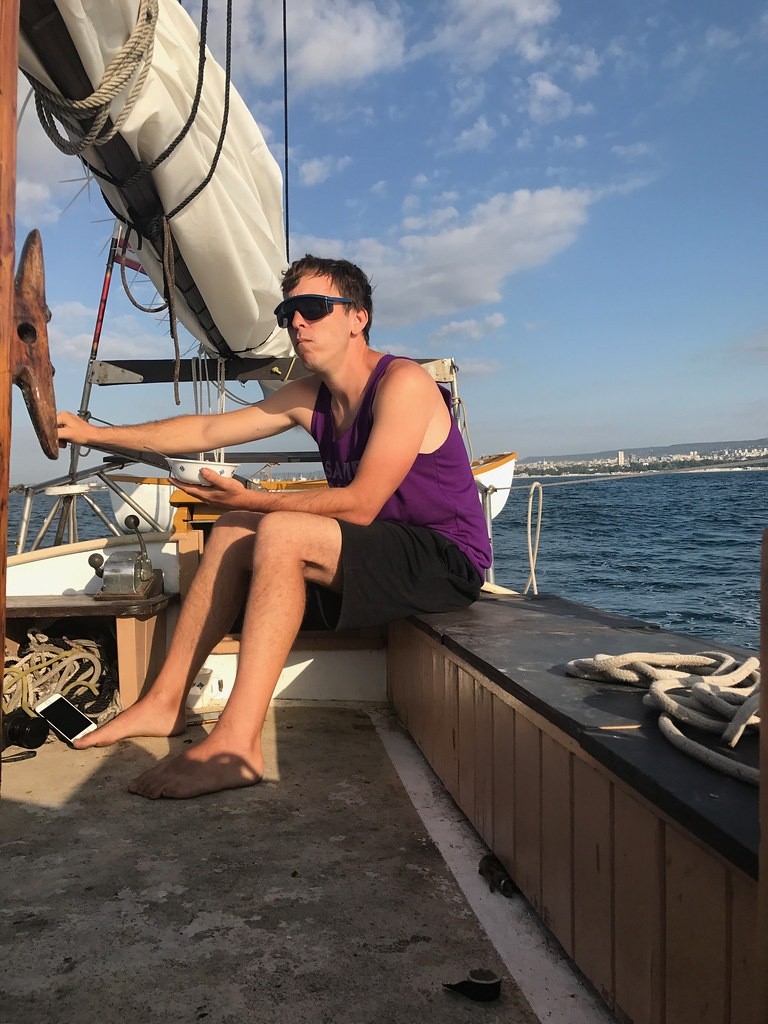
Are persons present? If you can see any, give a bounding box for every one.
[58,254,493,799]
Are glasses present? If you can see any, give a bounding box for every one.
[273,294,356,328]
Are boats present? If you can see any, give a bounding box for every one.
[103,451,520,536]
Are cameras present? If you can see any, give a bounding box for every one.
[2,703,50,754]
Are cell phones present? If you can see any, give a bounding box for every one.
[34,692,98,744]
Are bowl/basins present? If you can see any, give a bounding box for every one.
[164,457,240,486]
[185,667,213,708]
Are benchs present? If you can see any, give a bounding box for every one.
[4,591,181,709]
[387,592,759,1024]
